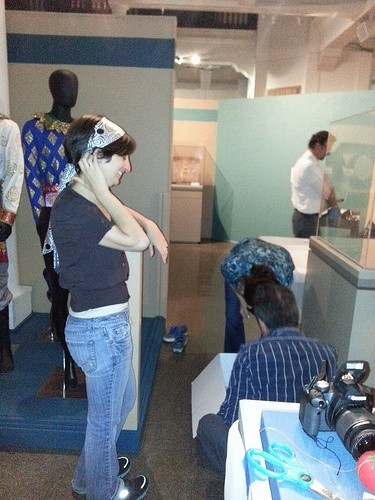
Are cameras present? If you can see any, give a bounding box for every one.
[299,360,375,462]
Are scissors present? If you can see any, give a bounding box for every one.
[246,442,344,500]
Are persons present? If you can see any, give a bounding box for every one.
[196,284,336,478]
[222,237,295,353]
[41,114,169,500]
[22,70,78,391]
[0,113,24,372]
[290,131,337,237]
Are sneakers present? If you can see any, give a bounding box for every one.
[162,324,189,353]
[71,456,149,500]
